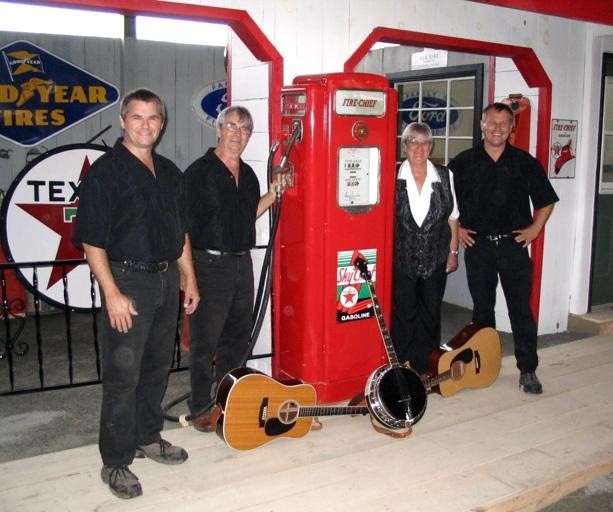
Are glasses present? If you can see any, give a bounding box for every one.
[406,139,430,145]
[225,123,251,135]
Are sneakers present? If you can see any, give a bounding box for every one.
[192,410,213,432]
[518,372,543,394]
[101,439,188,499]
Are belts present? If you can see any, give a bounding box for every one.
[110,259,171,273]
[487,234,509,241]
[192,248,249,256]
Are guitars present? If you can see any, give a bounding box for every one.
[352,257,427,428]
[347,324,501,417]
[215,367,372,450]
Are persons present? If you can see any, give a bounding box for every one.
[447,103,560,394]
[72,90,201,499]
[392,122,460,375]
[180,106,286,432]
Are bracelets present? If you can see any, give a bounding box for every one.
[450,250,459,254]
